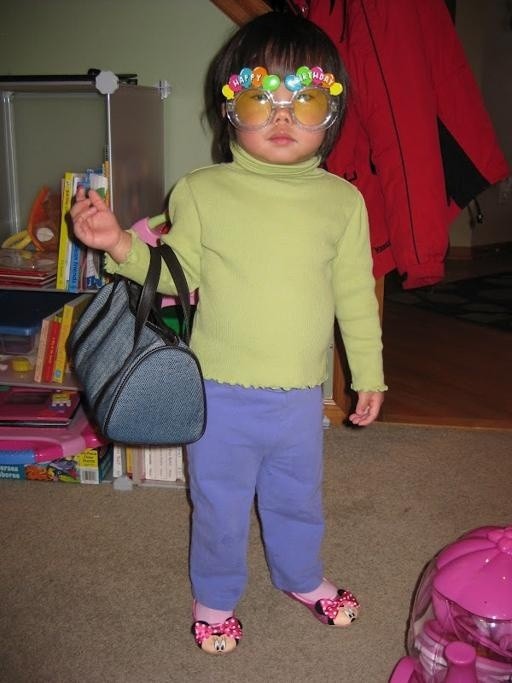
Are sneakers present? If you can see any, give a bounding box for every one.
[191,597,242,656]
[284,577,360,626]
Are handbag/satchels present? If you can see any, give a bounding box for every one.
[63,273,208,446]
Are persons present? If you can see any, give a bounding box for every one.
[68,5,390,653]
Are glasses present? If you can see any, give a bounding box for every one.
[220,66,344,133]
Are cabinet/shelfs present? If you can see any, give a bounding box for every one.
[0,71,167,484]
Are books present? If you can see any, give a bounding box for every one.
[57,160,112,290]
[33,294,96,385]
[113,440,186,483]
